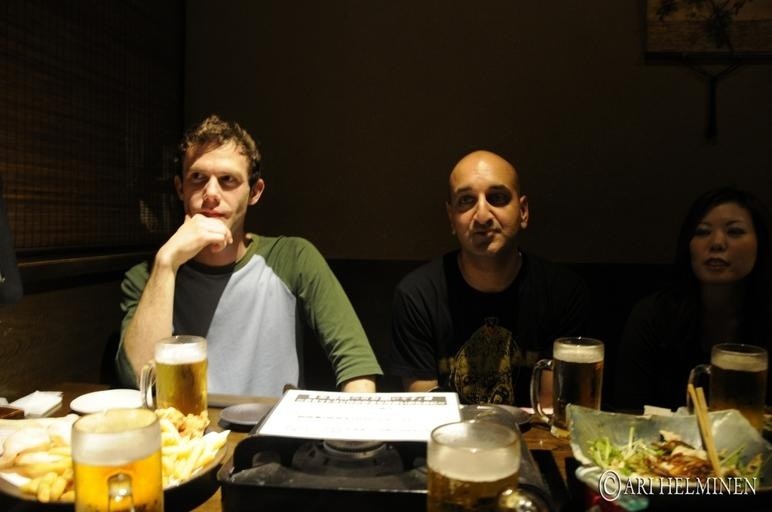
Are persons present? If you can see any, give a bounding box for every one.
[112,113,384,394]
[615,185,772,411]
[374,149,605,406]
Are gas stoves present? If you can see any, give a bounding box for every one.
[219,382,552,508]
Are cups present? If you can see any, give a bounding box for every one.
[526,334,606,442]
[140,334,209,430]
[70,409,164,512]
[686,339,770,439]
[422,418,537,512]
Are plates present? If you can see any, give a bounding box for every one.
[216,403,275,428]
[64,386,154,416]
[562,398,770,497]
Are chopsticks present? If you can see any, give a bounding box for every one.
[685,380,726,491]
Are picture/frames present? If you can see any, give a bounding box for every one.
[635,0,771,67]
